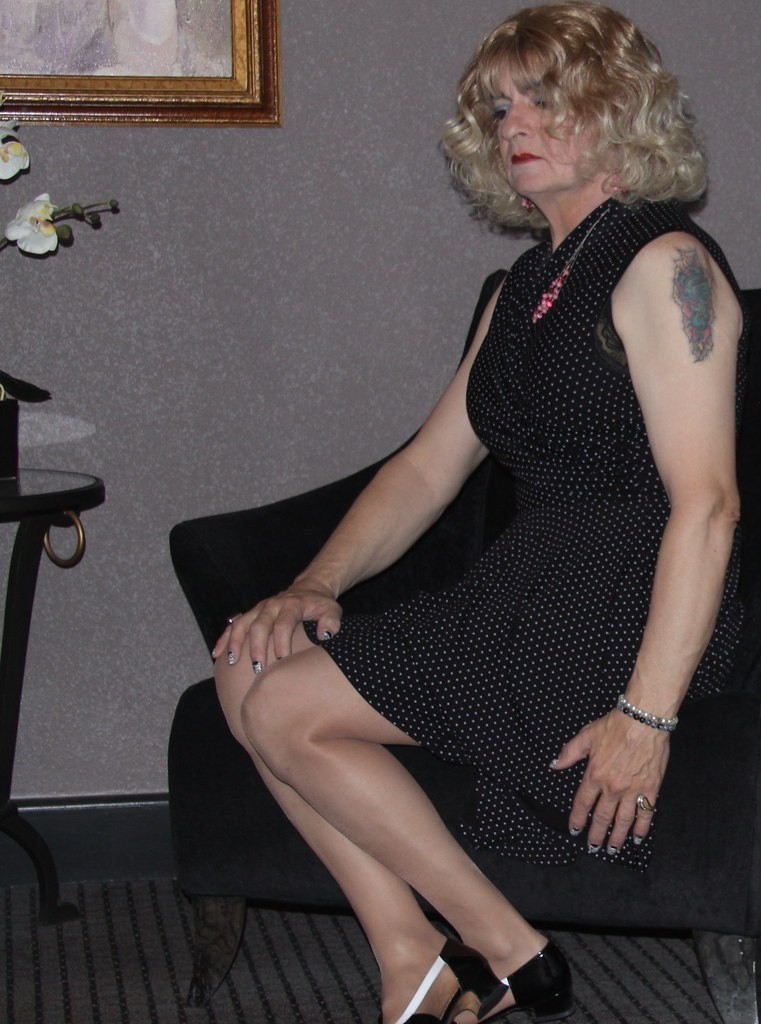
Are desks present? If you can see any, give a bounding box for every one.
[0,469,107,925]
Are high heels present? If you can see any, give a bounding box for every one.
[479,939,576,1024]
[394,938,506,1024]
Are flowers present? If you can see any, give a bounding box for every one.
[0,119,118,401]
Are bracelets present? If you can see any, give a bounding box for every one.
[617,695,678,731]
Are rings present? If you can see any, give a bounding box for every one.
[636,795,657,812]
[226,612,243,624]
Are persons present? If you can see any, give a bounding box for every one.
[211,0,761,1024]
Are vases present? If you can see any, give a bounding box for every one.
[0,399,20,481]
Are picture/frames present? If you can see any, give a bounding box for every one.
[0,0,282,129]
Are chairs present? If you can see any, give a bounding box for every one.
[166,288,761,1024]
